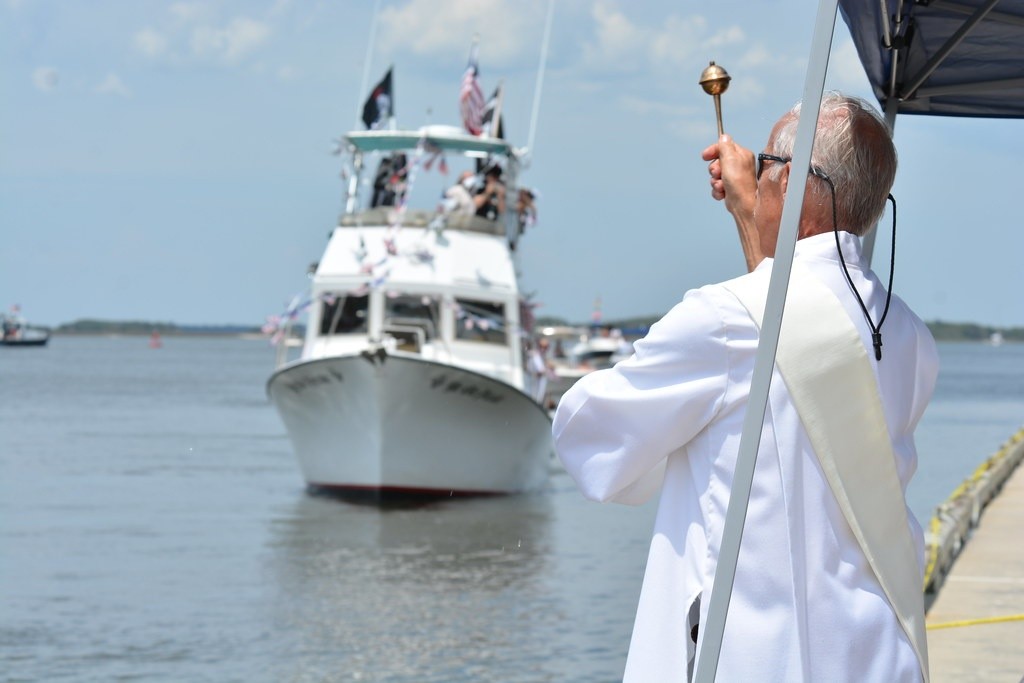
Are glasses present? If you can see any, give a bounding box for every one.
[757,153,831,180]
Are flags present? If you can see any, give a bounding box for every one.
[459,58,485,134]
[362,68,393,129]
[482,90,504,138]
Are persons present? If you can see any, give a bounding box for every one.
[550,94,939,683]
[528,339,556,379]
[453,165,535,224]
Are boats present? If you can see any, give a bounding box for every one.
[1,306,54,348]
[528,319,637,407]
[260,33,557,497]
